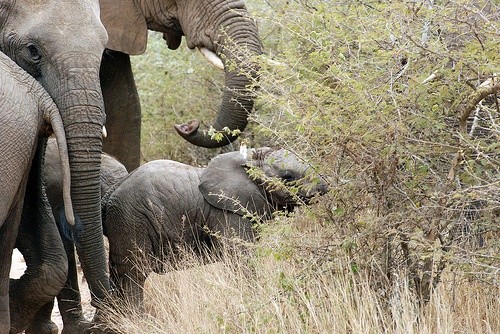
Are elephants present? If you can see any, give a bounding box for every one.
[100,147,330,310]
[0,0,261,334]
